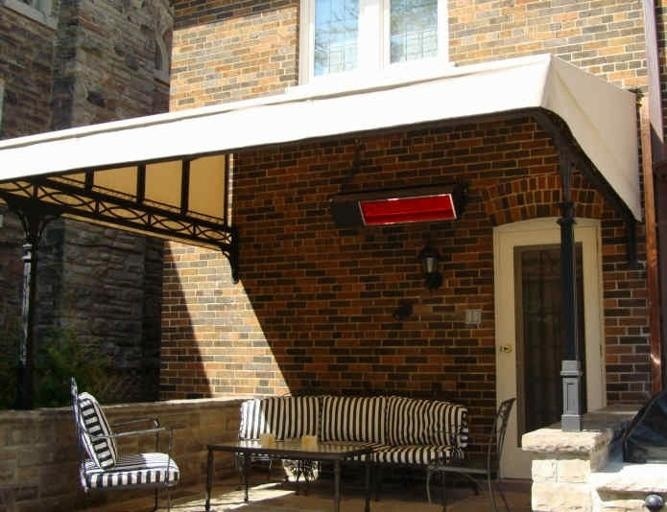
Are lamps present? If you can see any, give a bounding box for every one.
[326,178,469,229]
[418,246,439,275]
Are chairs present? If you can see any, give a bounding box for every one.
[422,397,516,512]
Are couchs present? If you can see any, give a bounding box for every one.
[70,377,180,512]
[234,395,470,497]
